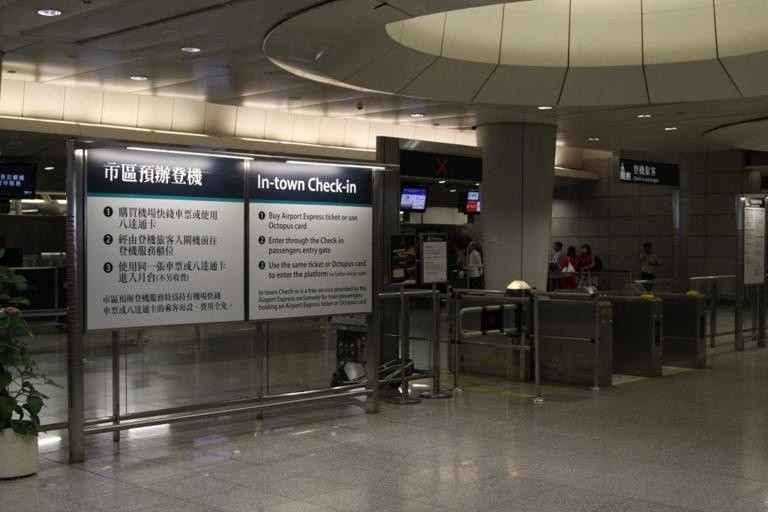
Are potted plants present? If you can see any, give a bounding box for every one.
[1,247,68,481]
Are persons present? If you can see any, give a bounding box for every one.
[466,239,482,289]
[550,241,564,272]
[576,244,594,281]
[639,242,660,292]
[557,246,578,290]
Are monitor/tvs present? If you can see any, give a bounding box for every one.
[464,191,482,215]
[458,191,464,213]
[400,184,429,212]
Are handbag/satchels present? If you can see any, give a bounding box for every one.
[560,262,577,278]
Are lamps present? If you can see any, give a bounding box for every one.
[504,280,532,298]
